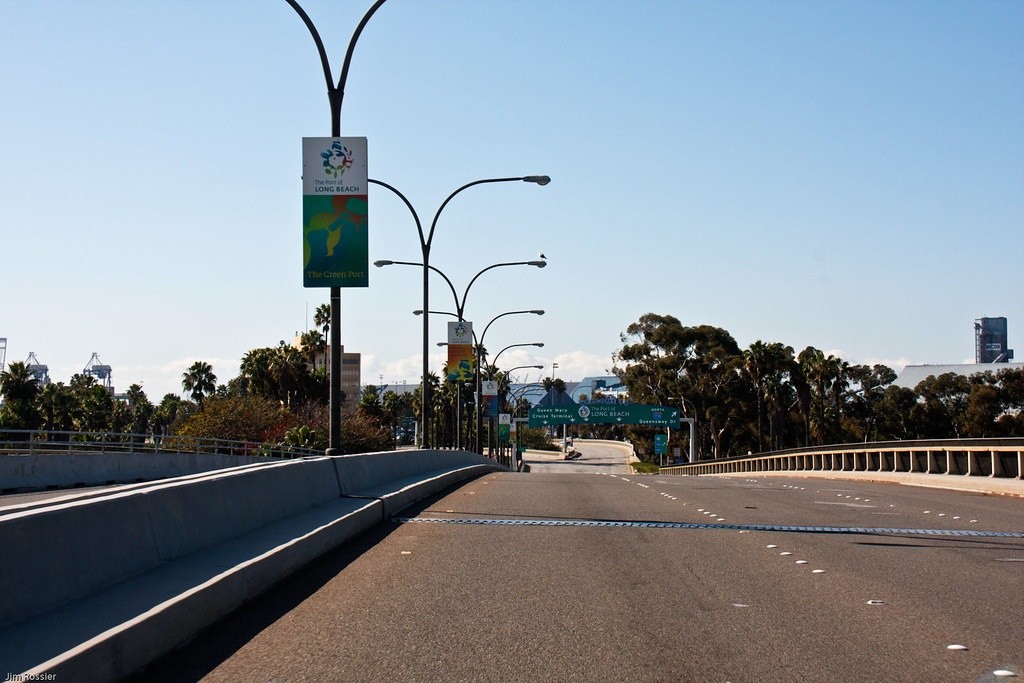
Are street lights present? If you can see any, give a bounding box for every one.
[442,366,545,464]
[364,175,552,450]
[668,397,698,462]
[414,308,546,456]
[373,259,548,452]
[436,342,546,460]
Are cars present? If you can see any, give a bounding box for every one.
[380,415,416,449]
